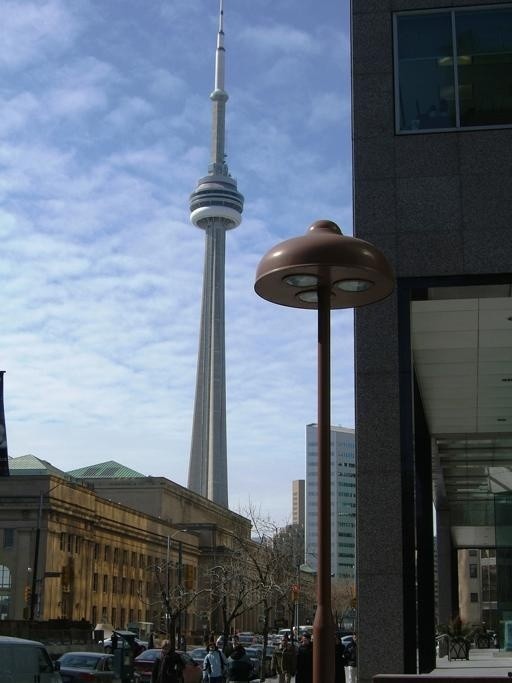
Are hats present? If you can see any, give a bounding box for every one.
[302,634,311,640]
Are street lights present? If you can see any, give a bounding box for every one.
[28,479,75,640]
[167,528,187,640]
[27,567,33,620]
[252,218,395,683]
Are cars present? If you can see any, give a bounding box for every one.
[188,625,356,679]
[52,651,142,683]
[98,633,148,652]
[0,635,64,683]
[132,648,203,683]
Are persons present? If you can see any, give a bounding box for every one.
[151,640,185,683]
[271,630,357,682]
[202,634,253,682]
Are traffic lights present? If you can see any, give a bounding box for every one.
[25,585,32,604]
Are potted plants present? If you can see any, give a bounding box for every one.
[436,613,482,662]
